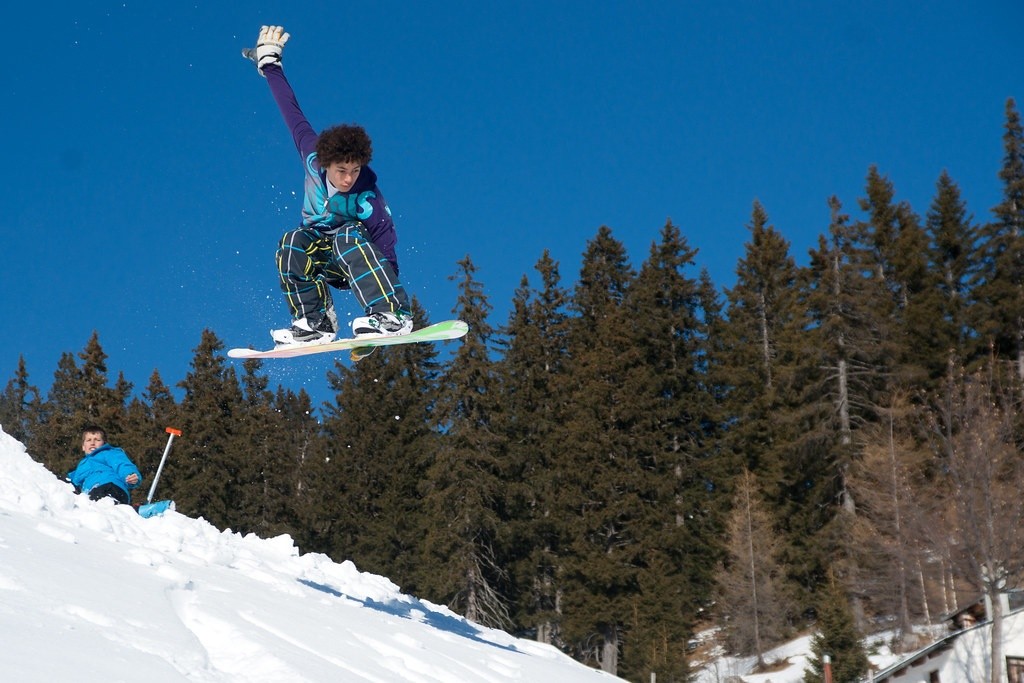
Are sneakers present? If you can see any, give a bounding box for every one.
[353,312,414,338]
[273,318,337,344]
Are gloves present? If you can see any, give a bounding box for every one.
[242,25,291,77]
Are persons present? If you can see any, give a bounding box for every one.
[244,24,413,346]
[63,426,142,505]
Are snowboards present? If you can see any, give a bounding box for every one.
[227,319,469,359]
